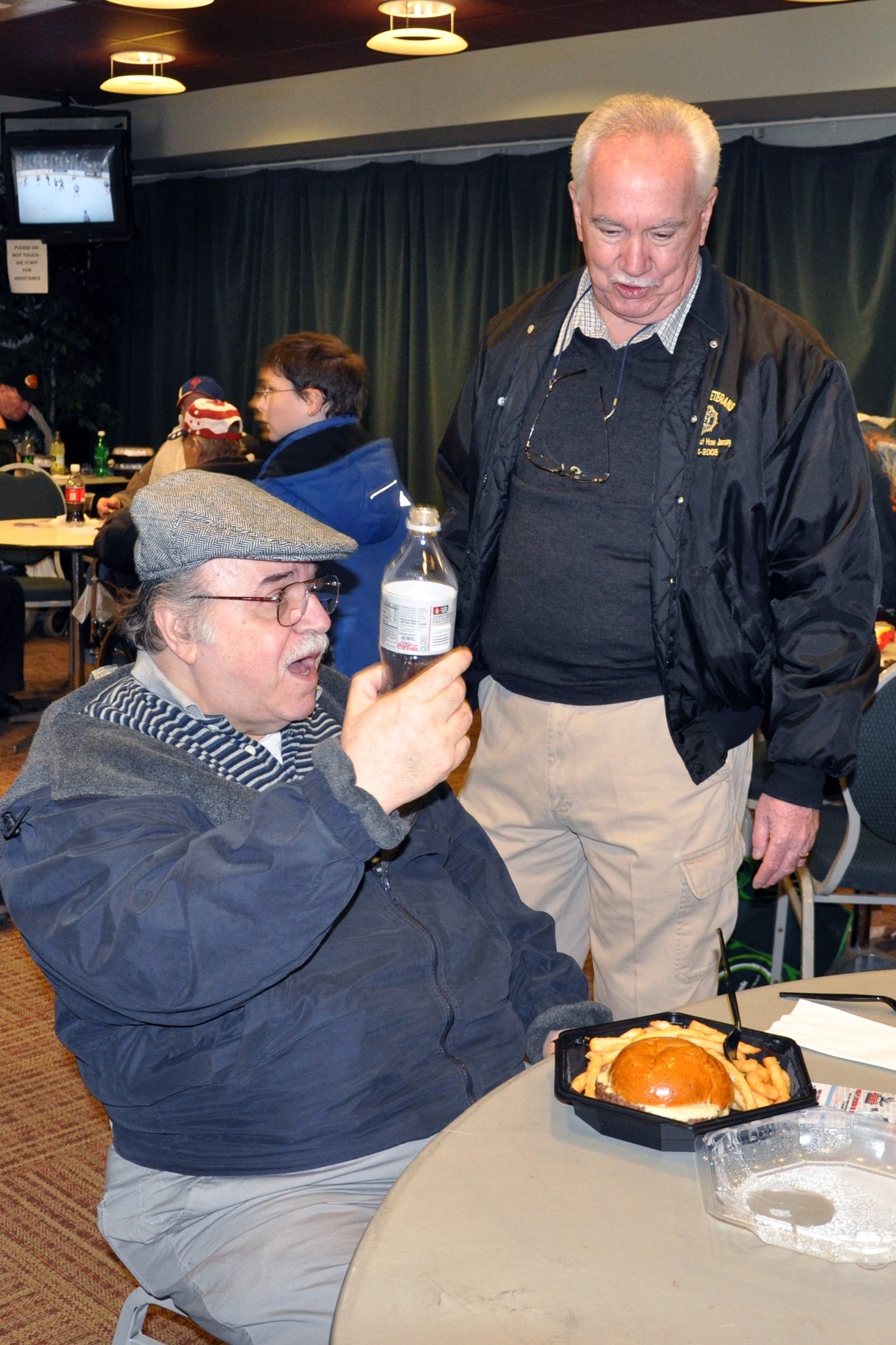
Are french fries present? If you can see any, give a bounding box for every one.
[569,1018,790,1112]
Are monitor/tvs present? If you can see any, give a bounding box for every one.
[1,129,129,242]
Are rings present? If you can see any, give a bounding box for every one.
[801,853,809,859]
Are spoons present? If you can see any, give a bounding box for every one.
[715,928,756,1071]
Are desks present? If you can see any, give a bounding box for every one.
[326,967,896,1345]
[55,472,131,485]
[0,518,105,690]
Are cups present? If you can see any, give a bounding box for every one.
[83,492,95,520]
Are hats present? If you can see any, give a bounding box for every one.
[0,369,41,404]
[167,397,242,440]
[177,375,220,405]
[131,468,359,581]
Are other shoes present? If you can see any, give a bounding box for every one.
[0,694,49,721]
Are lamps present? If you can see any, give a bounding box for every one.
[367,0,467,56]
[100,49,186,95]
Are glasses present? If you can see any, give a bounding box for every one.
[191,574,341,627]
[524,367,611,483]
[260,387,326,403]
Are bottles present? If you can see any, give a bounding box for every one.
[64,463,85,527]
[375,506,458,701]
[20,431,34,474]
[49,431,65,476]
[94,430,109,478]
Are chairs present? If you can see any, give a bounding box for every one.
[771,660,896,984]
[0,463,72,608]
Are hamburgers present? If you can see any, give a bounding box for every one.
[594,1035,734,1124]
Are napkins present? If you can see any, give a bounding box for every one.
[765,998,895,1073]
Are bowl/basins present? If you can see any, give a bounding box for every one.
[109,446,155,480]
[553,1011,896,1269]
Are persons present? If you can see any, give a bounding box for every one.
[423,89,882,1028]
[82,332,418,680]
[0,468,634,1345]
[0,363,55,477]
